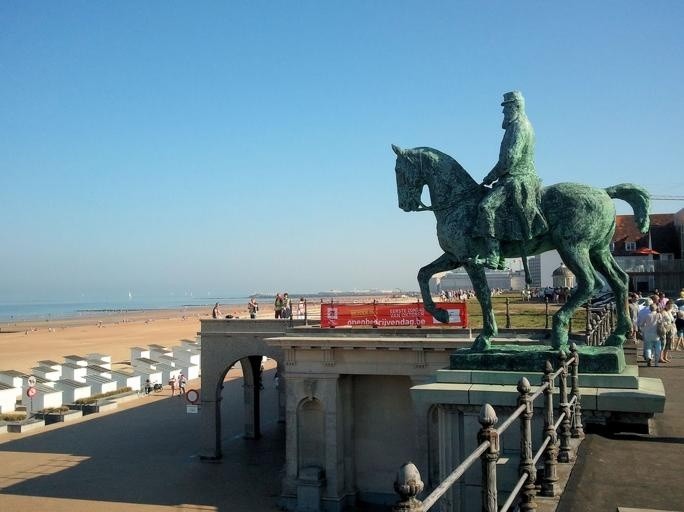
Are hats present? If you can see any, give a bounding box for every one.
[650,303,657,310]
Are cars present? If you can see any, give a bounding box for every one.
[541,284,684,322]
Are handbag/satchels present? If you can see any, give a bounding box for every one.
[656,315,666,337]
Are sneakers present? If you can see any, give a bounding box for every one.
[646,345,684,367]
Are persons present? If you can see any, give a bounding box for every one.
[178,372,184,379]
[282,292,291,318]
[247,295,260,320]
[438,285,578,307]
[466,89,550,271]
[274,292,283,319]
[178,375,187,398]
[210,300,223,319]
[628,286,683,366]
[167,374,177,400]
[297,297,305,316]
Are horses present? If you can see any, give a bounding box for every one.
[391,143,649,352]
